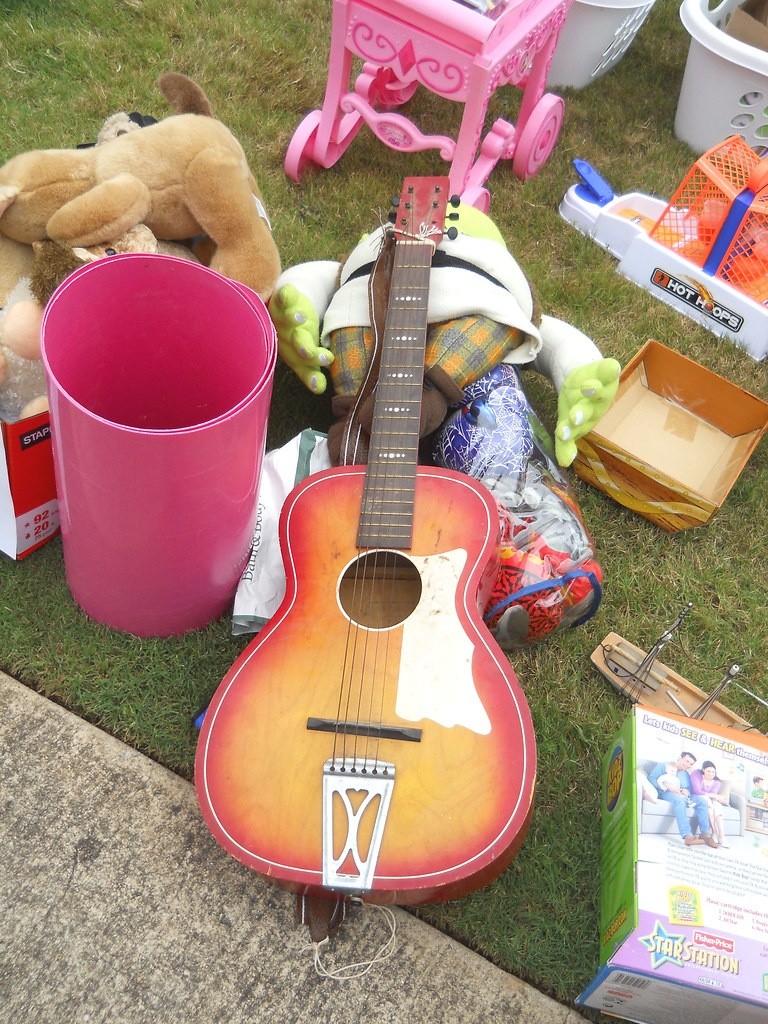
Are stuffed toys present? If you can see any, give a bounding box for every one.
[265,196,621,472]
[0,72,282,414]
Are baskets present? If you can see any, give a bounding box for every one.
[649,136,768,307]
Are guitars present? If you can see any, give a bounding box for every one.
[193,173,539,944]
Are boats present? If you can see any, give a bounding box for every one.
[590,601,768,737]
